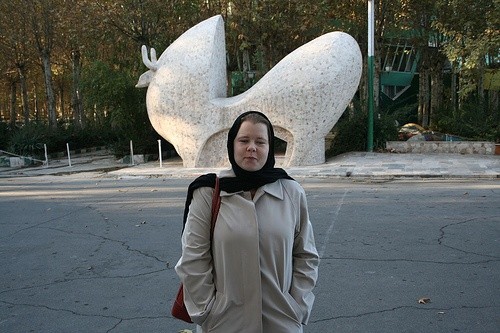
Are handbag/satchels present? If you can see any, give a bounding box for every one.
[171,282,194,324]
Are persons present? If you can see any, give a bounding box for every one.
[175,111,320,333]
